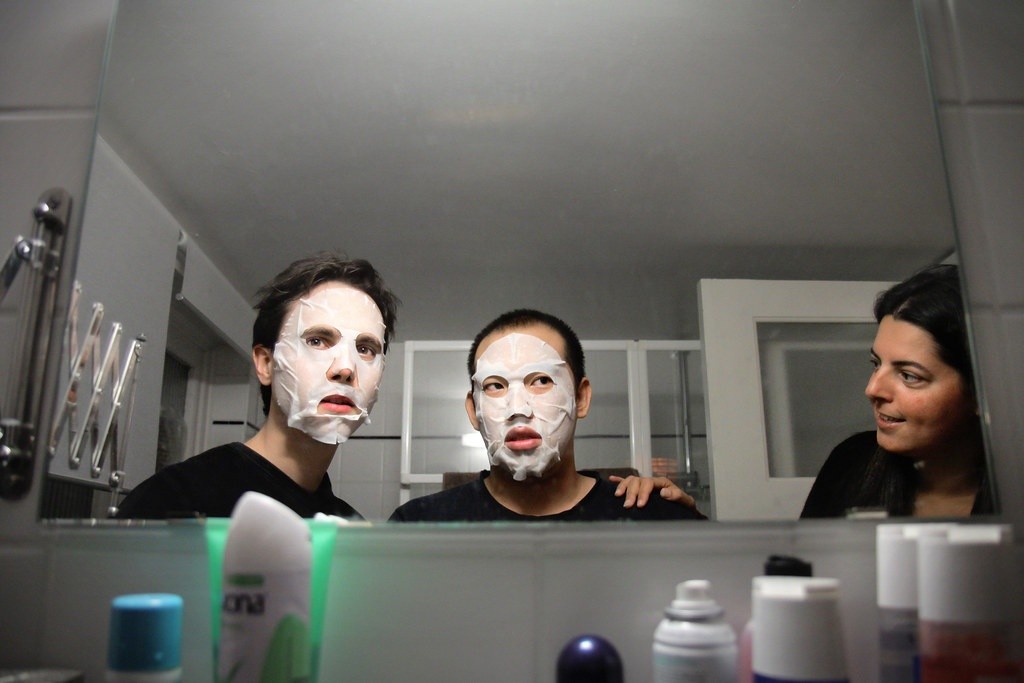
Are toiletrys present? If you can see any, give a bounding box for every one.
[554,521,1024,683]
[107,591,184,683]
[205,490,339,683]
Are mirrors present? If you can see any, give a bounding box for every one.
[29,0,1003,535]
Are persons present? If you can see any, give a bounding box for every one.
[113,254,398,521]
[384,310,708,521]
[610,263,993,521]
